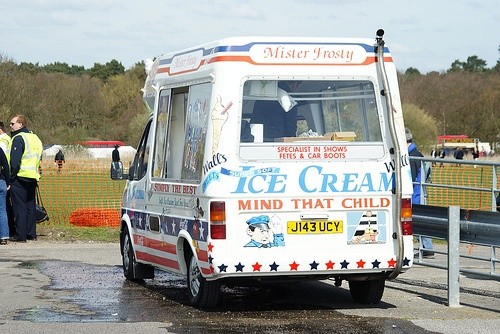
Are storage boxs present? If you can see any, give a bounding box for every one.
[279,132,357,141]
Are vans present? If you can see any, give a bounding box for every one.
[110,29,415,311]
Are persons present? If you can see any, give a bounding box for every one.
[111,144,121,162]
[0,114,66,245]
[241,80,302,142]
[430,147,479,168]
[406,128,435,258]
